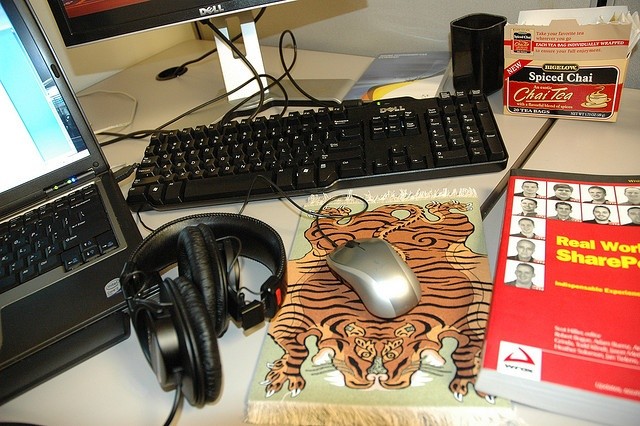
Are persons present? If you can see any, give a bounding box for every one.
[505,264,540,291]
[514,181,545,199]
[618,187,640,205]
[547,184,580,201]
[510,219,544,239]
[512,198,544,218]
[547,202,581,222]
[582,204,615,225]
[622,207,639,225]
[583,186,616,204]
[507,240,540,263]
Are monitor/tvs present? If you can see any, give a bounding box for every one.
[46,0,355,49]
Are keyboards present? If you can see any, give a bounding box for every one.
[125,89,509,213]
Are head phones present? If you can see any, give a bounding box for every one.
[119,212,287,407]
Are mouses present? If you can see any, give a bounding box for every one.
[326,238,422,320]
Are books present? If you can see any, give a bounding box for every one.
[341,51,454,101]
[474,169,639,426]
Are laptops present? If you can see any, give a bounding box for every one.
[1,1,144,407]
